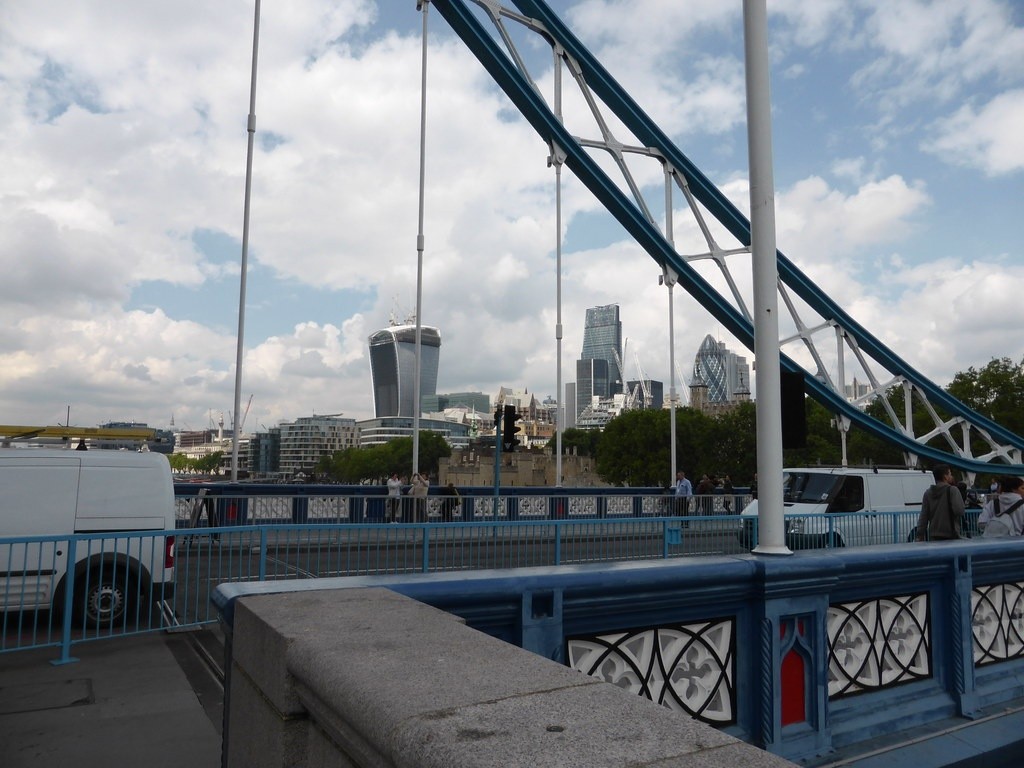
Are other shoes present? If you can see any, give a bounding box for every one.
[680,525,689,529]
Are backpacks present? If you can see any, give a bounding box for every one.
[981,498,1024,538]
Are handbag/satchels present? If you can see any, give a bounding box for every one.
[408,482,418,496]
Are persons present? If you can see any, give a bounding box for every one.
[675,470,757,529]
[387,471,460,526]
[828,463,1024,542]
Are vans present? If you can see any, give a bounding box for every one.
[0,433,179,629]
[732,466,939,551]
